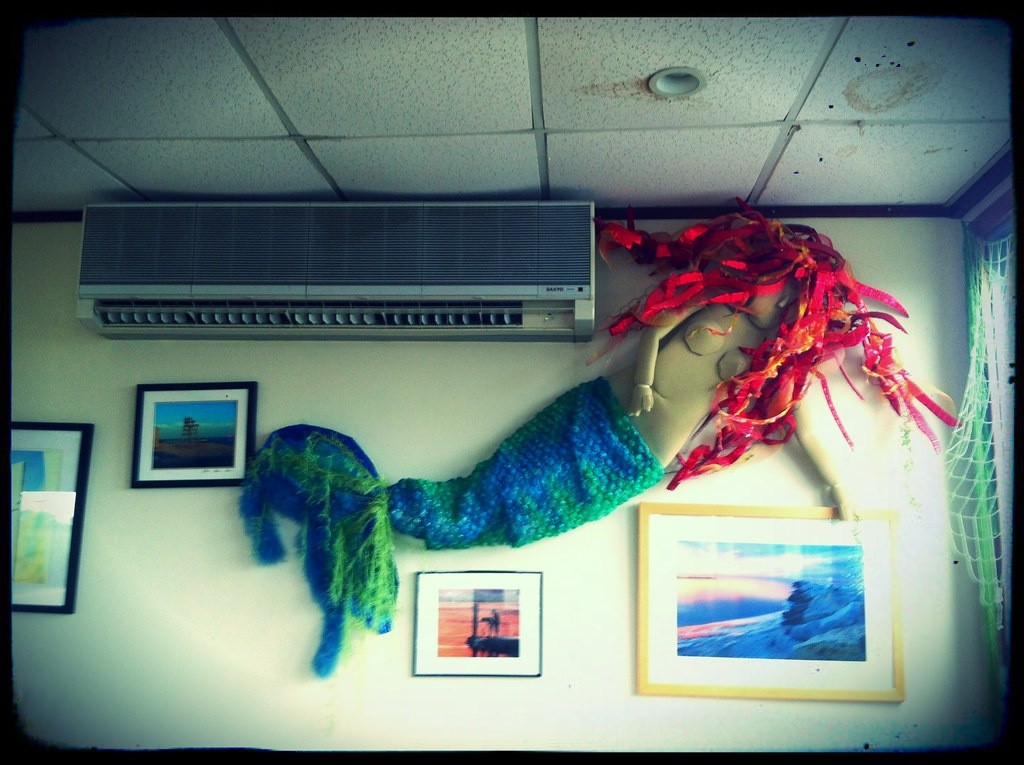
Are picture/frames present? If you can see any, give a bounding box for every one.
[412,570,542,677]
[10,421,95,616]
[638,502,904,702]
[131,380,257,489]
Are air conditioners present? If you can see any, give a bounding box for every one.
[76,198,600,341]
